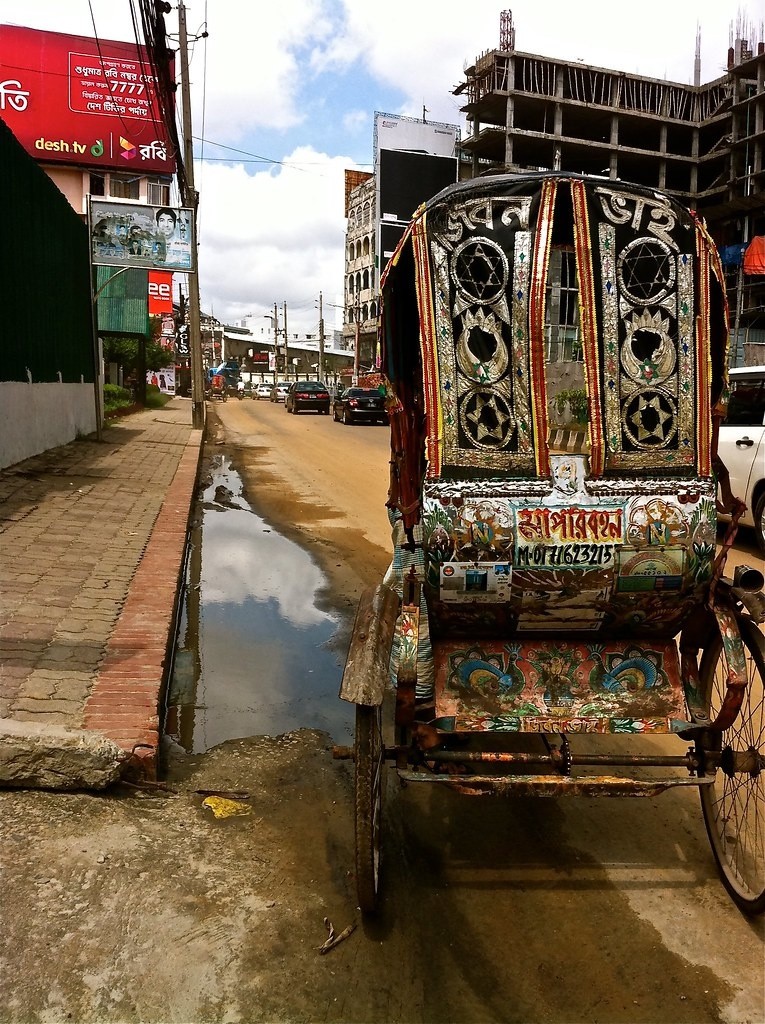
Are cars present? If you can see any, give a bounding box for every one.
[333,386,390,426]
[283,379,330,415]
[716,364,765,559]
[175,367,293,403]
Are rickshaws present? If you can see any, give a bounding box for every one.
[338,171,765,922]
